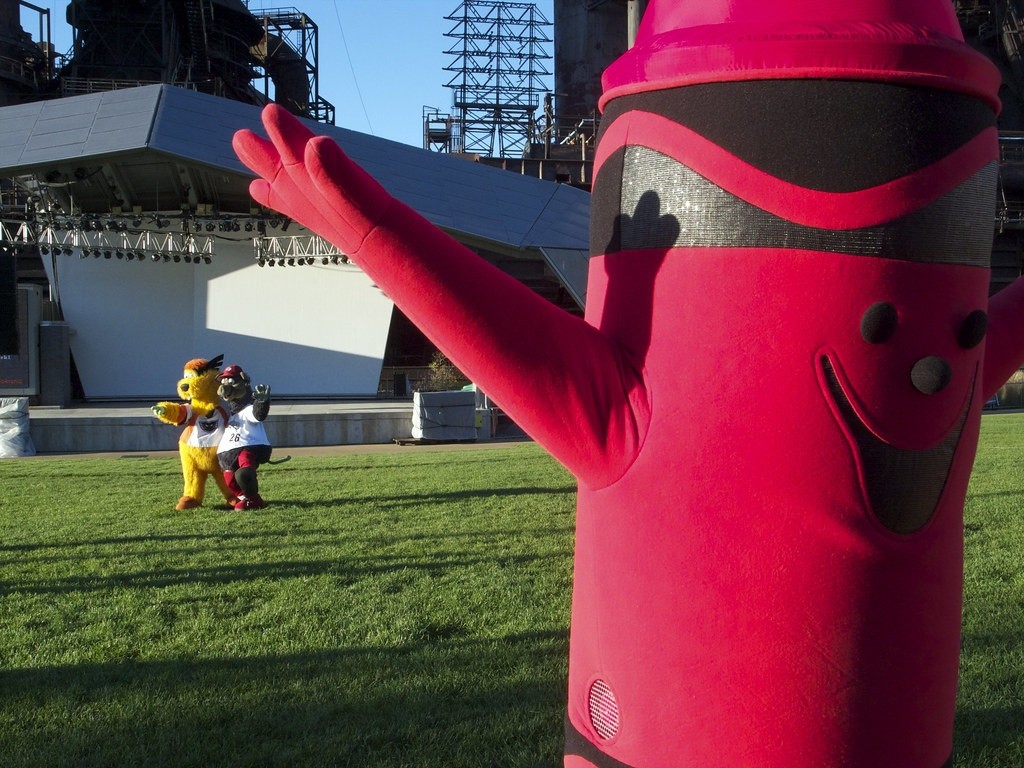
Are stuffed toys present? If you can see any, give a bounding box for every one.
[150,353,241,510]
[214,365,291,512]
[229,0,1023,768]
